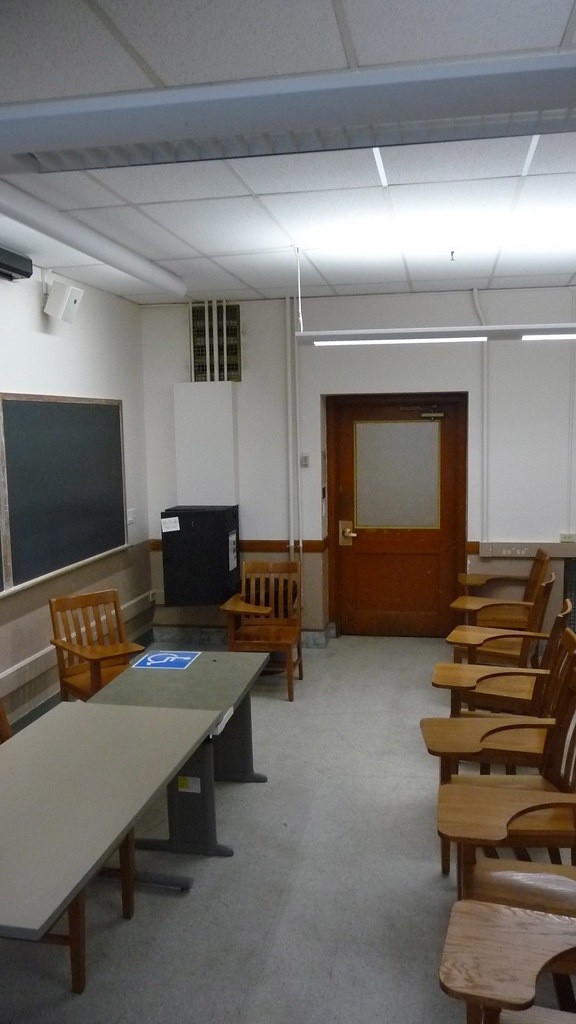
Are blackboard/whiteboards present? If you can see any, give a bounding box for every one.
[0,391,132,601]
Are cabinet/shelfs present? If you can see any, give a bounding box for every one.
[160,505,240,607]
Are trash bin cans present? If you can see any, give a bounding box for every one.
[235,577,298,676]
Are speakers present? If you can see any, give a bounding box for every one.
[44,281,84,324]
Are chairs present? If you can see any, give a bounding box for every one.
[420,547,576,1024]
[48,587,145,703]
[219,561,303,701]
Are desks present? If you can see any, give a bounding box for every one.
[450,596,534,613]
[458,573,529,587]
[220,593,272,615]
[421,718,556,757]
[438,899,576,1011]
[446,625,551,647]
[437,784,575,847]
[432,663,550,690]
[0,702,223,995]
[50,639,146,663]
[86,649,271,858]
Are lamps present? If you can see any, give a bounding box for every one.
[294,245,576,347]
